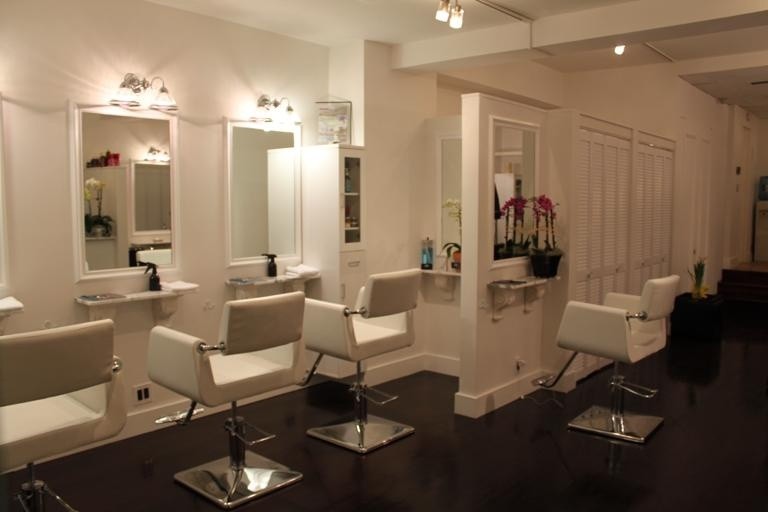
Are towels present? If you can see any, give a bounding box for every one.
[0,296,26,317]
[162,279,200,294]
[285,261,321,281]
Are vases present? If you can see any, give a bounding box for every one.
[528,248,563,279]
[452,250,462,264]
[495,245,527,261]
[89,226,110,239]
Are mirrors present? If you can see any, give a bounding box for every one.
[221,117,304,269]
[489,114,542,271]
[436,134,461,257]
[68,99,181,283]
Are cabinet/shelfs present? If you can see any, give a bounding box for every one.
[86,160,173,270]
[266,147,295,256]
[668,292,727,384]
[305,146,365,379]
[494,171,524,243]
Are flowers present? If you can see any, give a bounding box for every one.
[532,191,563,255]
[685,247,714,302]
[438,196,463,259]
[501,195,532,248]
[83,176,118,235]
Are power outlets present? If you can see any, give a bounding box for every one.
[514,355,523,378]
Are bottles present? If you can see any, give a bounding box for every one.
[421,236,434,270]
[91,150,120,167]
[344,168,352,193]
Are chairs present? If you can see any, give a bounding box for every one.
[143,289,310,511]
[296,267,424,455]
[528,273,684,445]
[0,318,130,511]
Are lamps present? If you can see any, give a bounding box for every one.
[110,72,180,113]
[432,0,467,30]
[247,92,305,125]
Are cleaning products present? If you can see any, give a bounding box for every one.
[143,261,161,291]
[263,251,277,278]
